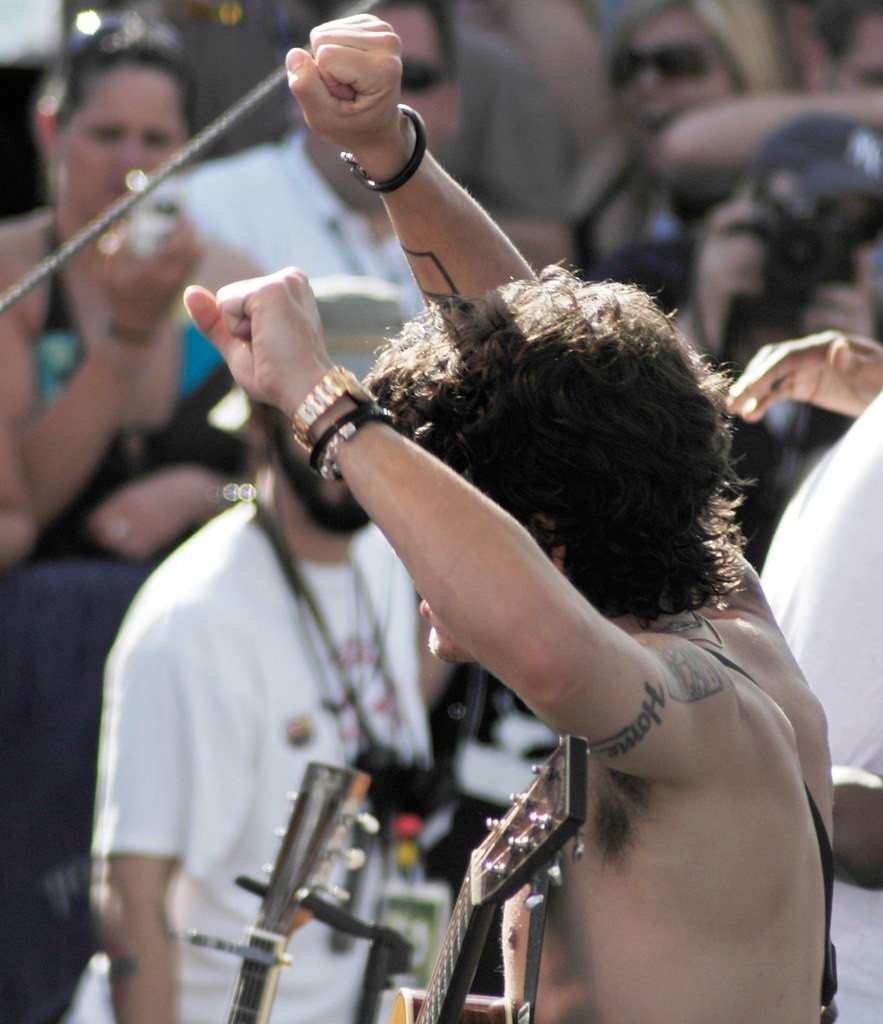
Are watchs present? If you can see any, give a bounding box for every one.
[290,363,377,453]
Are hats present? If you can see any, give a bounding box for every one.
[756,118,882,200]
[312,274,407,375]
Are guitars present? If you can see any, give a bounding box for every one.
[226,757,382,1024]
[388,730,596,1024]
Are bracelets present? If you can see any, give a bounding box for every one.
[306,407,382,482]
[320,406,403,482]
[338,104,428,194]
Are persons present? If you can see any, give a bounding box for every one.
[188,13,838,1024]
[0,0,883,1024]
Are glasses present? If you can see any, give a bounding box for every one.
[64,16,188,75]
[612,42,705,83]
